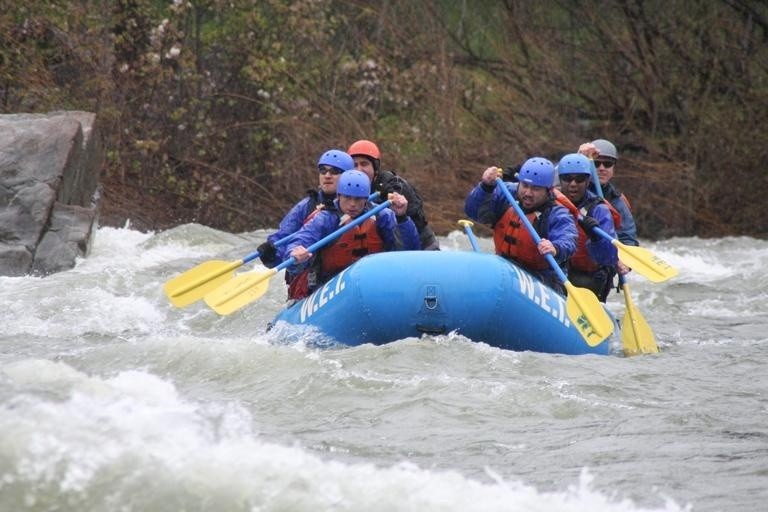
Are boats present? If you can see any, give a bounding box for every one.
[266,251,618,355]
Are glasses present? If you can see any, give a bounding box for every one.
[594,160,615,168]
[319,167,342,175]
[561,174,588,183]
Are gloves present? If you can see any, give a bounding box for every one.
[579,216,601,242]
[380,179,402,201]
[258,241,276,258]
[503,164,521,181]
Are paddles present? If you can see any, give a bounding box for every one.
[512,173,678,282]
[162,184,383,307]
[589,155,657,356]
[203,193,394,315]
[494,169,614,348]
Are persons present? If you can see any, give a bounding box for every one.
[290,169,424,307]
[463,156,578,303]
[576,138,637,290]
[257,149,381,283]
[346,139,442,256]
[500,152,620,309]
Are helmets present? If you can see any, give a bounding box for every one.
[338,169,370,200]
[592,139,618,160]
[318,150,354,170]
[348,140,381,165]
[558,154,591,180]
[519,157,554,189]
